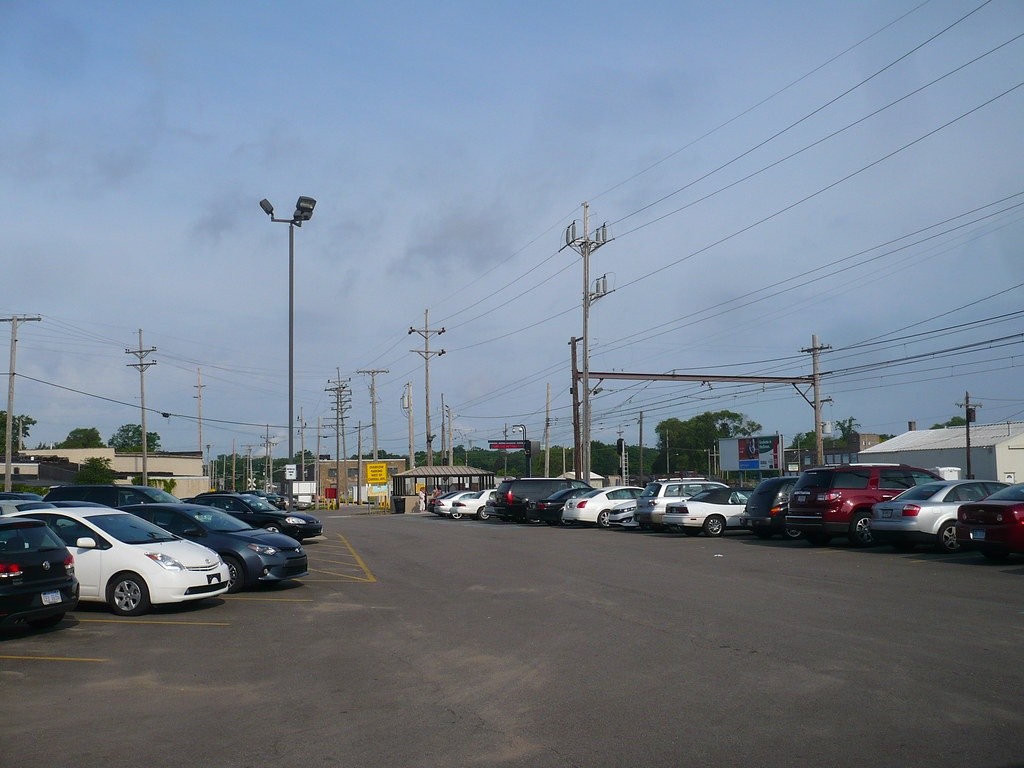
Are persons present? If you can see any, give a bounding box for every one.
[432,486,442,498]
[745,439,756,459]
[419,487,426,513]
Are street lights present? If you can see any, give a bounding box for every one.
[512,422,527,451]
[259,193,317,510]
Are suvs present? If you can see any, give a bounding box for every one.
[485,477,598,525]
[42,485,186,510]
[784,463,986,549]
[632,477,747,534]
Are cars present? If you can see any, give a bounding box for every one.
[427,490,457,516]
[178,489,312,511]
[955,482,1024,558]
[184,494,323,545]
[868,480,1011,555]
[0,505,231,618]
[661,487,753,538]
[0,488,114,519]
[739,476,805,540]
[449,488,498,520]
[607,498,641,530]
[112,504,310,594]
[533,488,602,527]
[561,485,653,529]
[434,490,493,519]
[0,515,81,638]
